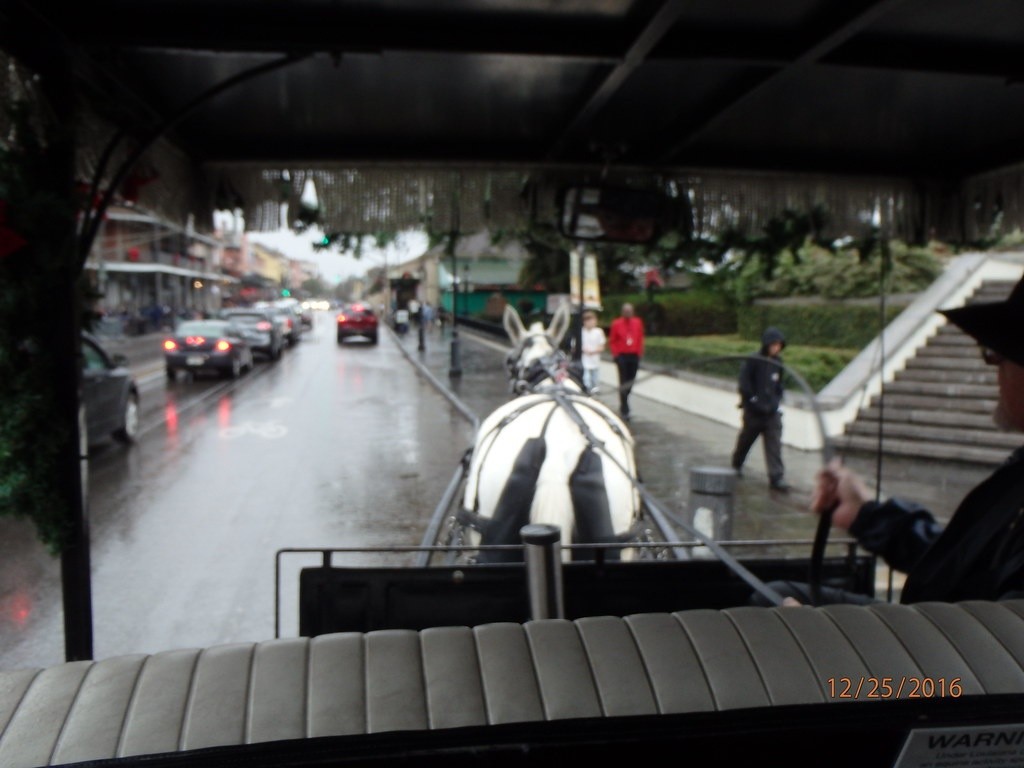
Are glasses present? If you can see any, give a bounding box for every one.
[981,347,1009,365]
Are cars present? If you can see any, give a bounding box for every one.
[251,296,315,347]
[337,301,380,344]
[217,306,284,363]
[80,330,141,450]
[163,317,254,378]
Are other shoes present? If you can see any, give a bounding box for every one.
[622,411,630,420]
[732,462,743,478]
[769,476,791,492]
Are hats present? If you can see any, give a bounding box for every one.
[937,272,1024,367]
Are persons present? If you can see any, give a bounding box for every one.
[570,312,607,394]
[783,274,1024,608]
[408,297,449,335]
[732,327,794,493]
[610,302,644,414]
[645,261,663,301]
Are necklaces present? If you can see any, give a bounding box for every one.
[626,338,633,347]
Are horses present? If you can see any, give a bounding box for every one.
[461,298,643,564]
